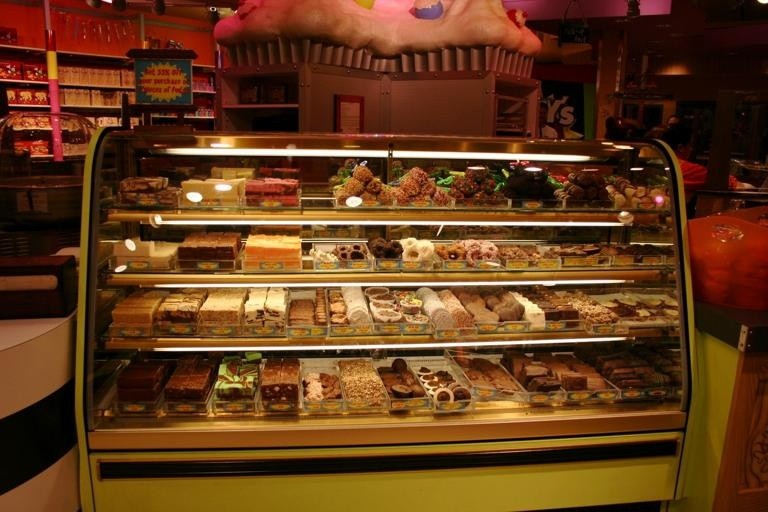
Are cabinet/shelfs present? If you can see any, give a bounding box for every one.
[73,127,700,512]
[216,65,310,132]
[0,44,217,161]
[484,73,542,136]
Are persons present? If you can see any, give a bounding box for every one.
[658,129,755,204]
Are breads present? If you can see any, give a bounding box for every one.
[565,289,620,335]
[163,354,220,415]
[412,364,471,410]
[393,166,452,207]
[199,288,250,335]
[247,180,299,207]
[461,291,525,332]
[305,368,340,414]
[245,288,291,335]
[556,170,613,207]
[448,166,508,207]
[331,287,371,334]
[366,282,428,334]
[436,239,499,260]
[110,289,166,335]
[603,292,679,332]
[413,288,472,338]
[529,287,578,332]
[156,288,207,335]
[454,354,519,398]
[242,234,302,271]
[311,242,371,268]
[500,348,617,407]
[116,357,178,414]
[369,232,432,262]
[336,166,392,206]
[214,353,265,414]
[178,232,246,269]
[377,359,427,410]
[502,165,560,209]
[339,360,384,414]
[289,287,327,337]
[114,239,181,270]
[586,350,680,401]
[606,177,669,210]
[183,178,247,209]
[117,176,181,208]
[262,358,302,415]
